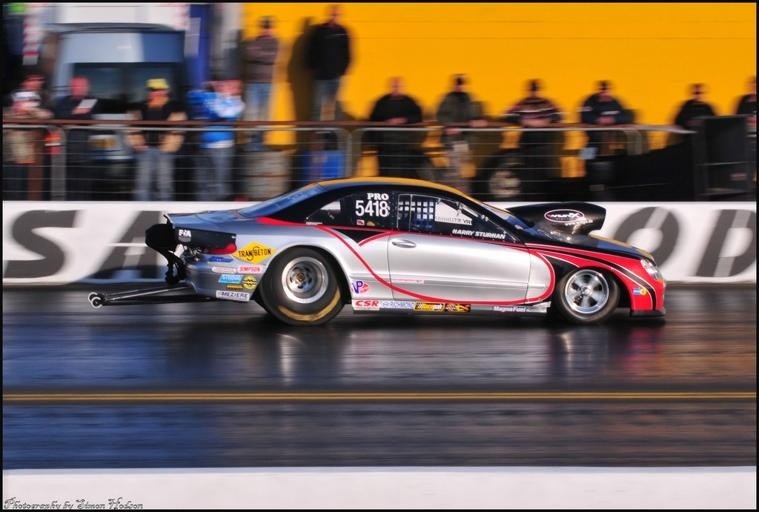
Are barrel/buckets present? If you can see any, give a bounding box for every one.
[188,89,242,150]
[238,143,297,200]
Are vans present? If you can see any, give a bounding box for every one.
[38,23,183,106]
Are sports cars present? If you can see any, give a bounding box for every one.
[145,176,665,326]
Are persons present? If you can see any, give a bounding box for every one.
[0,0,758,201]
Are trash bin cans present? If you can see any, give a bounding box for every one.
[235,142,345,202]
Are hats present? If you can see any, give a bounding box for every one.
[144,78,169,90]
